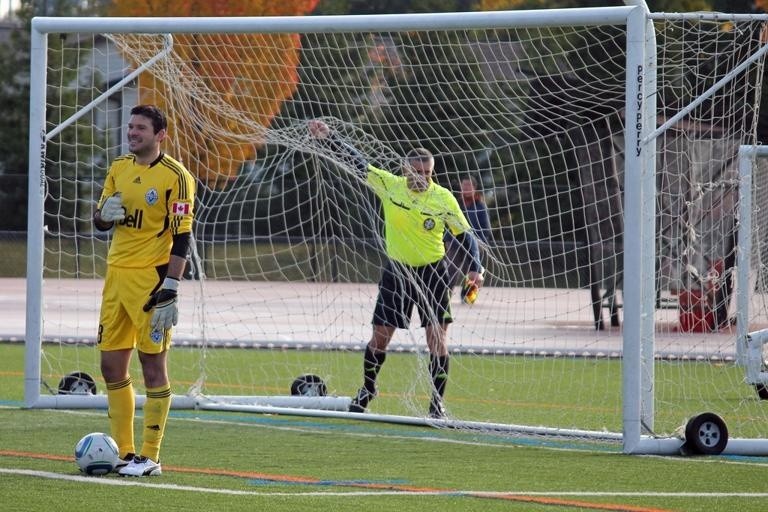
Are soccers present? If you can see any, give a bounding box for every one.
[75,432,121,477]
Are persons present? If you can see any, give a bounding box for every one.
[308,118,484,422]
[442,174,493,298]
[94,105,194,477]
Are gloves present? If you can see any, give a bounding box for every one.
[100,196,125,222]
[143,276,179,333]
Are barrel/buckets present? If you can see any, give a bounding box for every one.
[680,289,714,333]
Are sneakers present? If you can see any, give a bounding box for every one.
[429,401,448,420]
[349,386,377,412]
[111,458,135,474]
[118,455,161,477]
